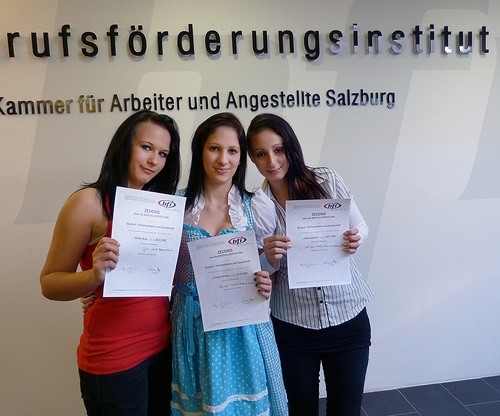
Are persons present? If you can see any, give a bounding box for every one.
[246,112,376,416]
[81,113,291,416]
[39,109,182,416]
